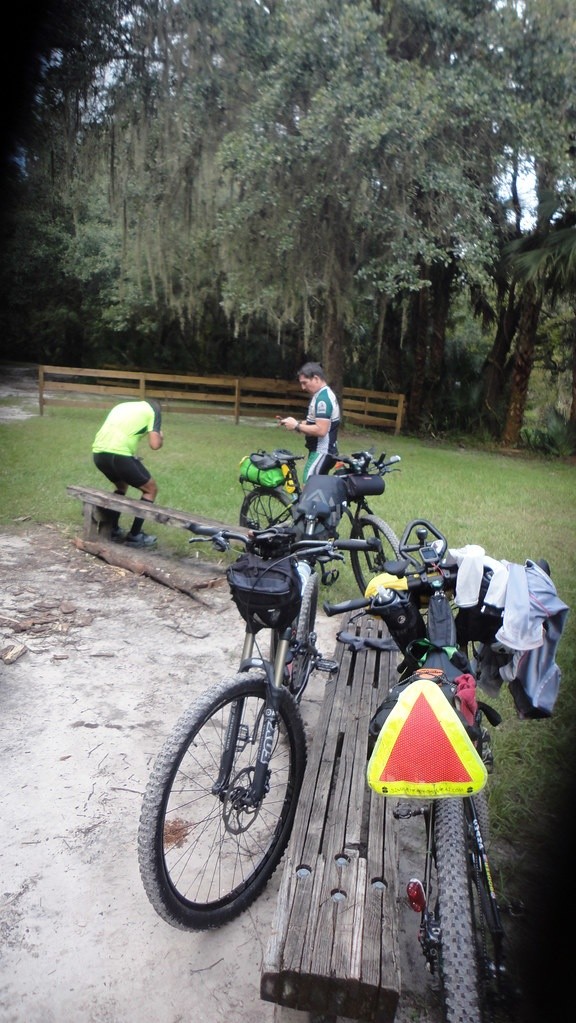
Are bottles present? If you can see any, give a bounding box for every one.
[292,557,312,597]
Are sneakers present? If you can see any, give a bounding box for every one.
[126,532,157,546]
[98,527,129,543]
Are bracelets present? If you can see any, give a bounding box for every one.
[299,421,302,424]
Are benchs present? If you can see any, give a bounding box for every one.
[65,483,262,543]
[258,595,402,1022]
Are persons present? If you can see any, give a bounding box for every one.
[93,400,164,548]
[280,363,340,493]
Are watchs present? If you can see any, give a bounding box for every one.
[295,423,300,433]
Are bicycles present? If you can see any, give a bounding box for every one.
[234,446,405,594]
[136,525,384,937]
[317,518,565,1021]
